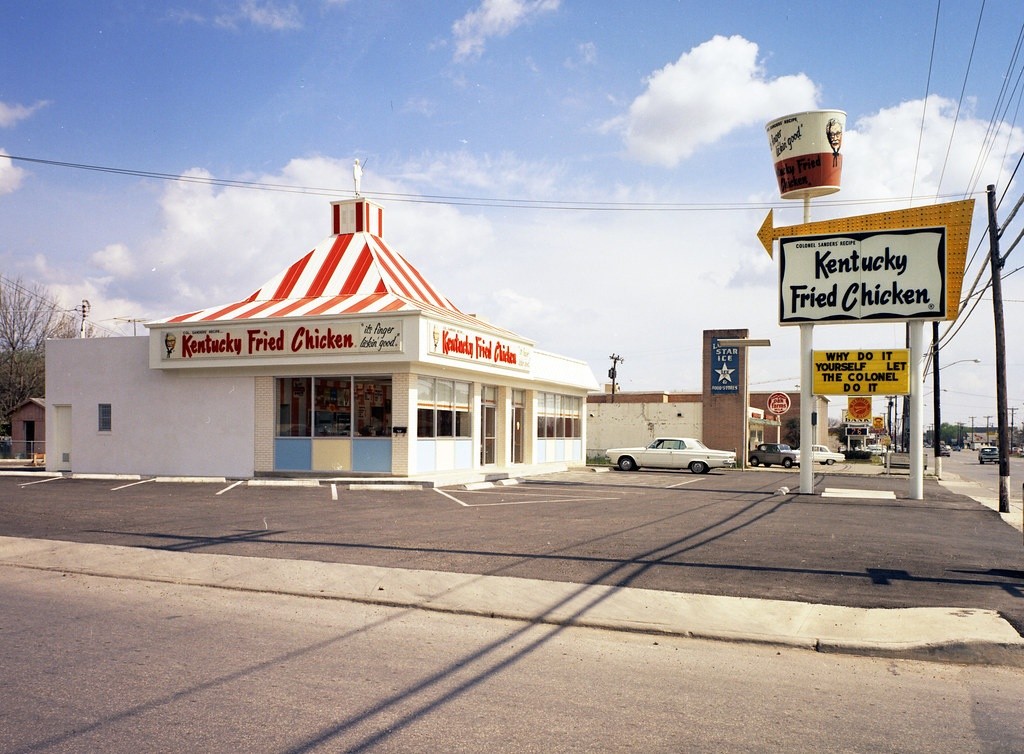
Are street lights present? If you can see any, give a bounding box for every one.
[923,389,948,397]
[923,403,928,407]
[1008,407,1023,454]
[968,416,977,442]
[983,415,994,446]
[924,359,982,378]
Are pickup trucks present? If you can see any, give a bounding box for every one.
[796,445,845,465]
[748,443,800,468]
[978,447,999,464]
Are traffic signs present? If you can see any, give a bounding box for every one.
[779,225,947,323]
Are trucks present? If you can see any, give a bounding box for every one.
[940,449,951,457]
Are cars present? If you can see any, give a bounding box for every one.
[953,444,961,451]
[605,438,737,474]
[925,444,931,448]
[892,445,895,450]
[1010,447,1022,451]
[898,446,901,452]
[946,445,951,450]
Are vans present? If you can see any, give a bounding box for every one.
[867,448,886,456]
